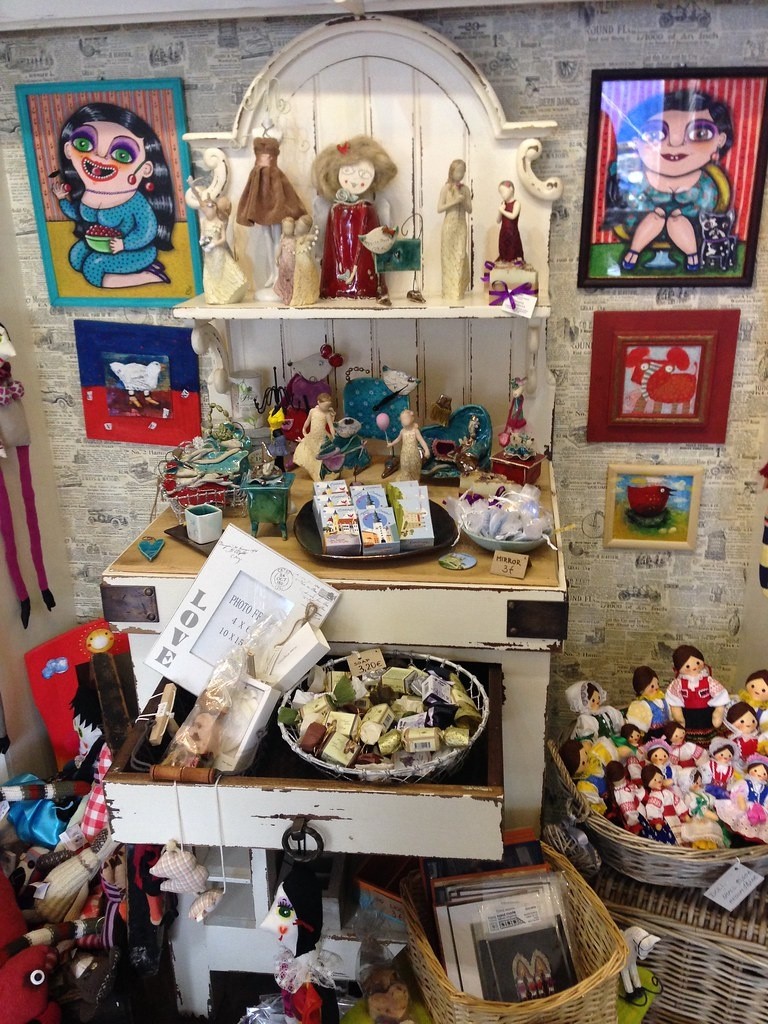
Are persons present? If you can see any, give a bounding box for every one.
[197,199,249,303]
[559,645,768,851]
[235,119,310,289]
[503,376,530,435]
[494,180,525,262]
[252,442,282,480]
[449,414,482,472]
[264,405,289,473]
[0,682,106,843]
[384,409,430,482]
[436,158,474,300]
[273,214,320,303]
[258,864,340,1024]
[292,395,339,483]
[312,133,394,301]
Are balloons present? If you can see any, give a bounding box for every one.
[376,413,390,431]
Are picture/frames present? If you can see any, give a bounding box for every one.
[601,464,704,552]
[607,330,719,430]
[13,75,204,309]
[142,520,344,699]
[576,64,768,289]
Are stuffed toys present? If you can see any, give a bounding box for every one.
[181,687,231,767]
[0,743,165,1024]
[153,846,223,922]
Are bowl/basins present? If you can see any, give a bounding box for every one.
[461,510,552,552]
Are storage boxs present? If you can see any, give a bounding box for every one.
[309,478,435,556]
[210,671,283,775]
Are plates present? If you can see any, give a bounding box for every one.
[293,495,460,560]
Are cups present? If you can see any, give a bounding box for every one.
[185,504,222,544]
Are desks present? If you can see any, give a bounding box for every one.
[105,458,571,1024]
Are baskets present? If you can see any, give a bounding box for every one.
[548,719,768,888]
[400,838,630,1024]
[584,861,768,1024]
[277,649,490,786]
[163,479,249,525]
